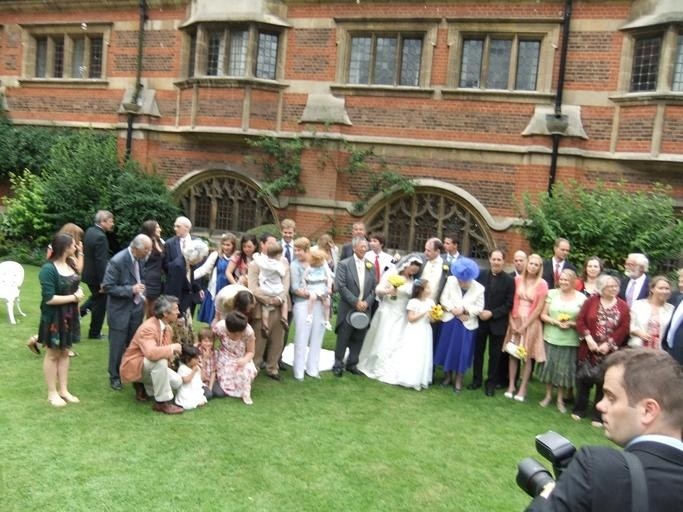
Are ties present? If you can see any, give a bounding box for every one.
[448,257,455,262]
[666,300,683,348]
[284,244,291,263]
[183,240,186,248]
[553,263,560,288]
[626,280,637,308]
[162,329,168,344]
[133,260,141,305]
[375,255,380,283]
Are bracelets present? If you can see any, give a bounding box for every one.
[73,293,79,302]
[77,251,83,256]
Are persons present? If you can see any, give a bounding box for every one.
[332,222,683,426]
[26,223,86,357]
[36,235,87,407]
[79,209,116,338]
[106,216,338,414]
[519,348,682,510]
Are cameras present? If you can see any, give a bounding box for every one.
[178,343,199,357]
[516,430,577,500]
[133,292,147,306]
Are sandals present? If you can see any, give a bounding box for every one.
[261,324,272,339]
[60,392,79,403]
[322,321,332,330]
[280,317,289,331]
[27,337,41,354]
[47,396,67,406]
[68,351,78,357]
[306,314,313,325]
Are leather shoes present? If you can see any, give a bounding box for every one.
[111,378,121,390]
[455,385,461,393]
[485,381,495,396]
[279,366,286,371]
[514,392,526,401]
[153,401,184,414]
[539,399,549,407]
[133,382,149,401]
[88,335,107,341]
[555,401,566,413]
[345,365,360,375]
[440,377,452,389]
[466,384,482,390]
[268,371,281,380]
[333,366,342,377]
[504,387,517,398]
[496,384,508,389]
[80,307,87,317]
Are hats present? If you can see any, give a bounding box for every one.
[345,308,370,330]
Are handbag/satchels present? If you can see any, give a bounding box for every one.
[505,341,525,359]
[576,361,605,384]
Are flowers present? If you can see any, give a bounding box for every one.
[442,265,450,271]
[557,310,572,324]
[428,306,444,324]
[514,336,526,364]
[386,272,408,301]
[365,262,372,272]
[389,252,400,264]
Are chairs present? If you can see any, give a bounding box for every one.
[0,257,29,326]
